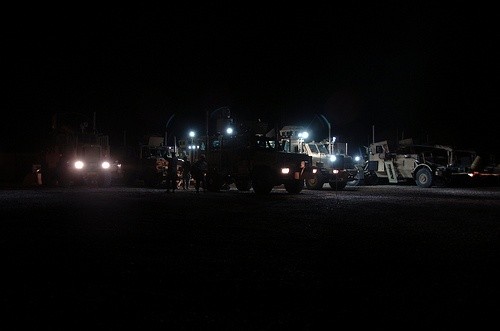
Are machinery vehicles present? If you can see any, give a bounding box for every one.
[65,131,454,195]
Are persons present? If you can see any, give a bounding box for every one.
[194,154,208,193]
[182,156,190,190]
[163,151,180,191]
